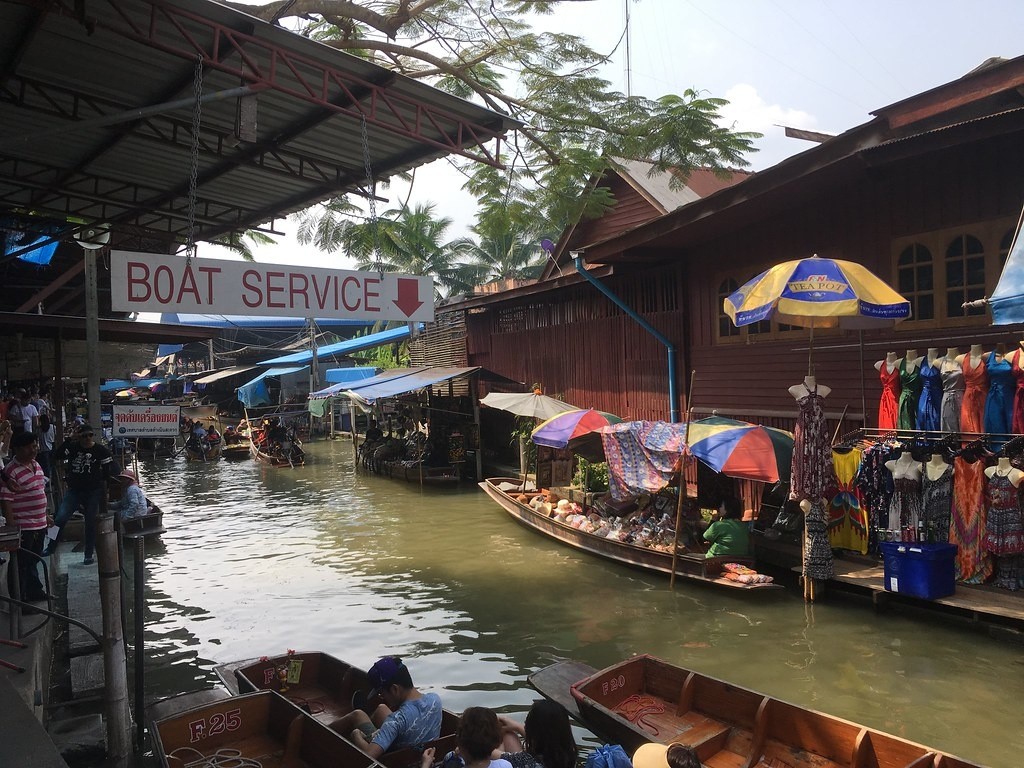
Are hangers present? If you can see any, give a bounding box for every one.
[833,429,864,450]
[869,429,897,454]
[963,436,990,462]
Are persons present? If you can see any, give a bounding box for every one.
[0,377,89,471]
[979,458,1024,557]
[0,431,55,615]
[0,469,22,492]
[180,418,248,460]
[121,436,136,452]
[685,496,749,559]
[266,421,286,446]
[917,454,955,544]
[41,425,120,565]
[106,470,148,535]
[874,340,1024,453]
[325,657,701,768]
[359,420,383,447]
[885,451,922,542]
[787,375,840,503]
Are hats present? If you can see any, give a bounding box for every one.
[118,470,135,481]
[78,425,93,433]
[241,418,247,425]
[632,742,685,768]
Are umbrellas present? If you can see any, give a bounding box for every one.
[684,410,795,485]
[115,384,166,400]
[530,406,625,451]
[722,253,915,375]
[479,389,582,495]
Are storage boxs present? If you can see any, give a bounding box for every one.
[881,541,958,598]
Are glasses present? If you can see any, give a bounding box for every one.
[378,681,400,700]
[81,432,93,438]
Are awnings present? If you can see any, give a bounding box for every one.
[193,365,260,384]
[307,365,481,486]
[989,220,1024,324]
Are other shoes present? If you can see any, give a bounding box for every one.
[41,546,55,556]
[31,590,54,602]
[352,690,373,716]
[84,557,94,565]
[22,607,39,616]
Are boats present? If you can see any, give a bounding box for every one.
[137,438,176,461]
[110,476,167,539]
[142,686,385,768]
[222,439,251,457]
[244,402,309,467]
[180,403,223,461]
[526,652,990,768]
[477,477,785,599]
[213,650,461,768]
